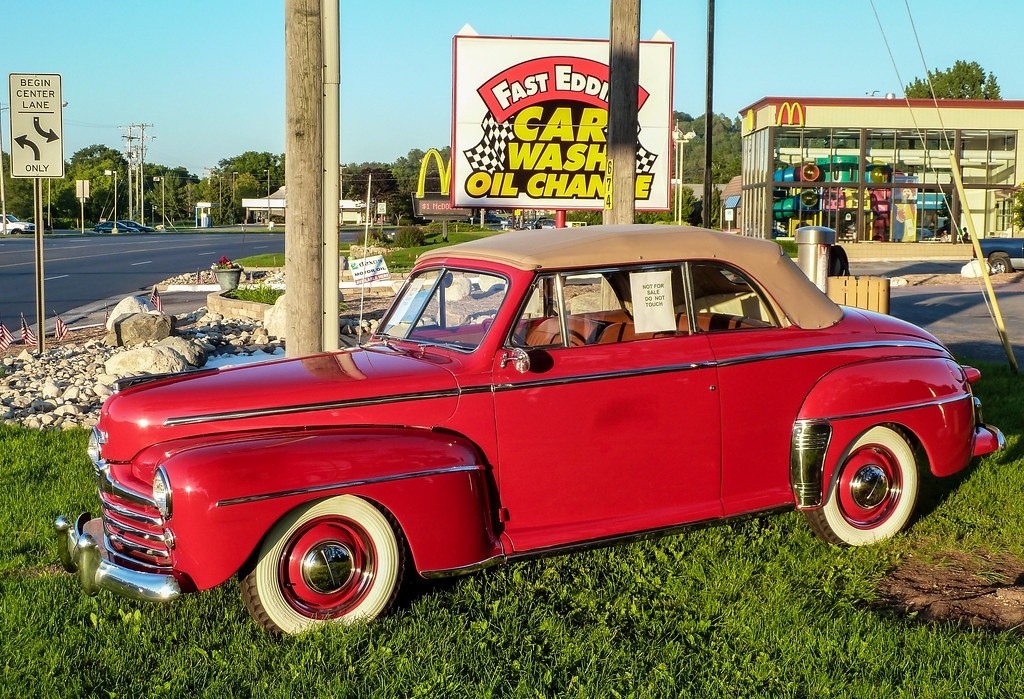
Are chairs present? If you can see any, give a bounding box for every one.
[598,322,682,345]
[517,317,605,348]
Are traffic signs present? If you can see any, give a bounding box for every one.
[8,72,66,179]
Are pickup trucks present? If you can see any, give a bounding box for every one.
[973,237,1024,276]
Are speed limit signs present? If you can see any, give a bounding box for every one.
[378,203,386,214]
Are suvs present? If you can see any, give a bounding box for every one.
[0,214,35,235]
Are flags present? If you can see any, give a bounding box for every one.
[0,321,16,352]
[21,315,38,346]
[55,314,69,342]
[150,286,164,313]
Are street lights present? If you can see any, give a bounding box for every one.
[104,170,119,234]
[0,100,68,236]
[153,176,166,232]
[672,127,696,225]
[232,172,239,227]
[264,170,270,229]
[340,166,347,226]
[219,177,225,225]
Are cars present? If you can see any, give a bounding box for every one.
[458,213,519,225]
[55,224,1007,642]
[92,221,140,235]
[521,216,568,230]
[117,220,156,233]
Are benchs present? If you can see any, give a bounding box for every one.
[602,308,763,332]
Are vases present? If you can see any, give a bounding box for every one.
[213,269,244,290]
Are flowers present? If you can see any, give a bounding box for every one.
[214,256,239,269]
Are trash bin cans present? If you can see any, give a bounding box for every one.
[793,225,836,295]
[200,213,211,227]
